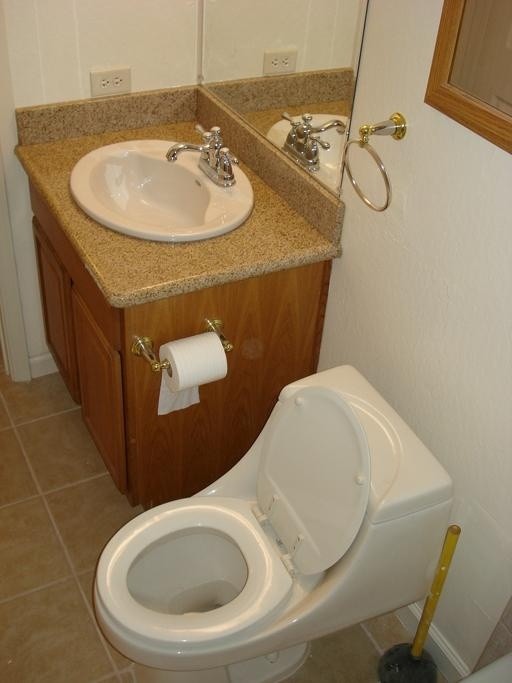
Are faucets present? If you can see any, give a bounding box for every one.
[282,112,346,172]
[166,125,239,186]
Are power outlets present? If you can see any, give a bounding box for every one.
[262,50,298,74]
[90,65,131,97]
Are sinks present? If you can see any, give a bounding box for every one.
[266,114,351,192]
[69,139,255,241]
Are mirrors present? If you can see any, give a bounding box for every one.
[199,0,370,200]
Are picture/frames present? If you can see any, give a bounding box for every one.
[423,0,512,156]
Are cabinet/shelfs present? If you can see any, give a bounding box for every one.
[26,174,332,512]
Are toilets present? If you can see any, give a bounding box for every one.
[93,363,454,682]
